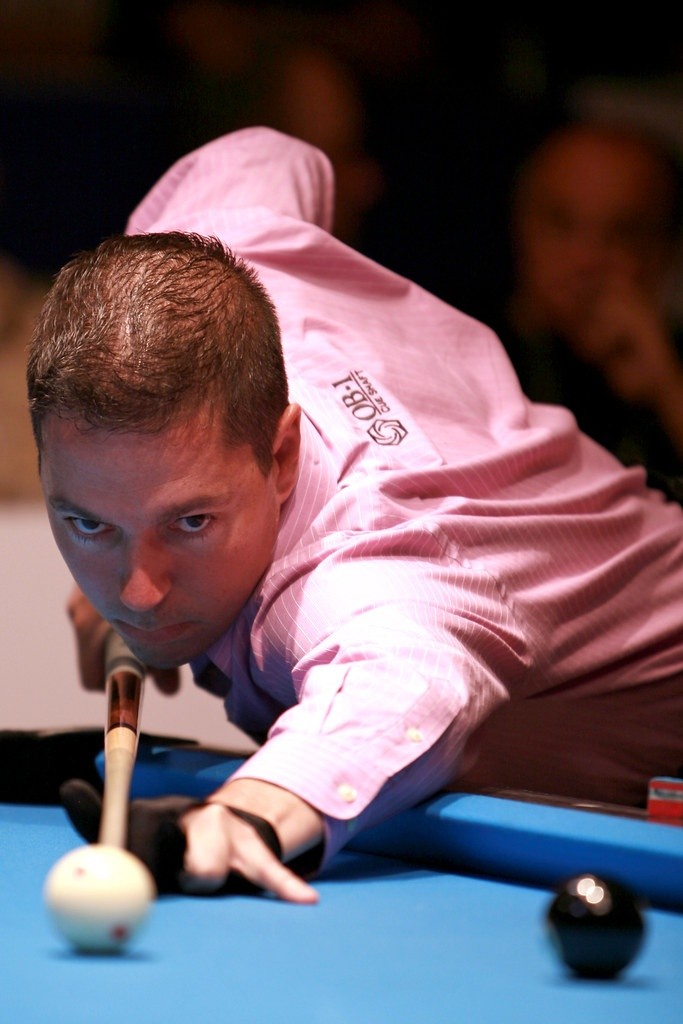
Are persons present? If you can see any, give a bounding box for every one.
[28,124,682,901]
[0,1,682,501]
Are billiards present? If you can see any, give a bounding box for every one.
[42,841,148,954]
[548,873,647,979]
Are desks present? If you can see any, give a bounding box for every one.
[0,727,683,1024]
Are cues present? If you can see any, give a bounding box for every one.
[94,629,145,852]
